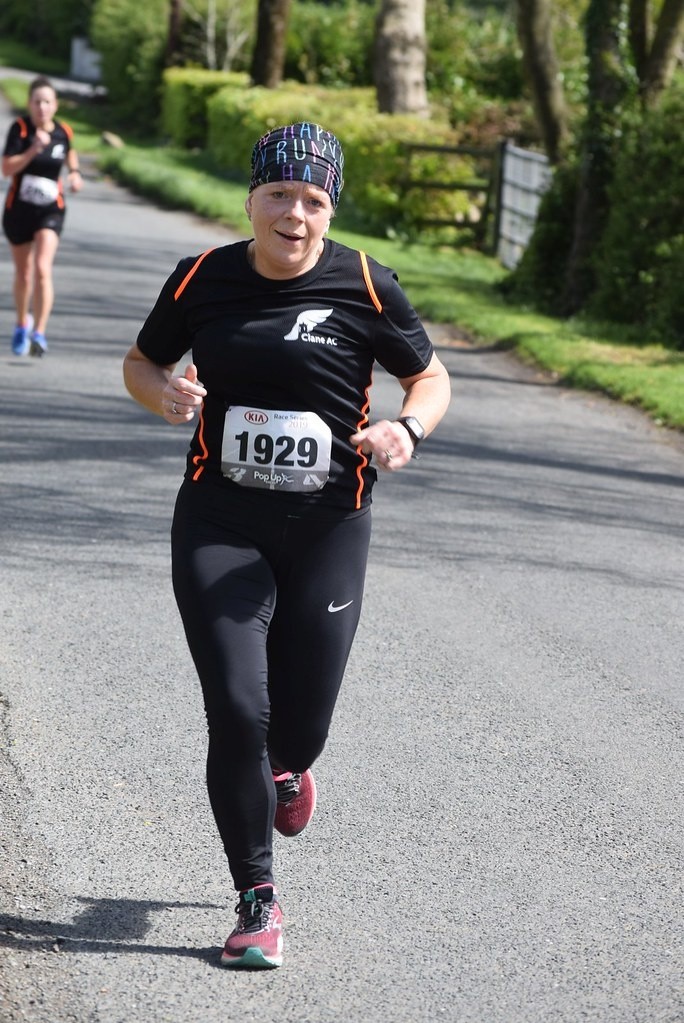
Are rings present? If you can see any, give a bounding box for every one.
[172,403,177,414]
[384,450,393,460]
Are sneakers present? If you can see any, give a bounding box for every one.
[11,313,34,356]
[30,331,49,357]
[220,883,284,968]
[271,769,317,839]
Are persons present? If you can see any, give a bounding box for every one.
[0,77,83,360]
[122,122,452,968]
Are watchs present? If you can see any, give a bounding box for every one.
[396,416,425,460]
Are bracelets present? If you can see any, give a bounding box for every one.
[69,168,80,174]
